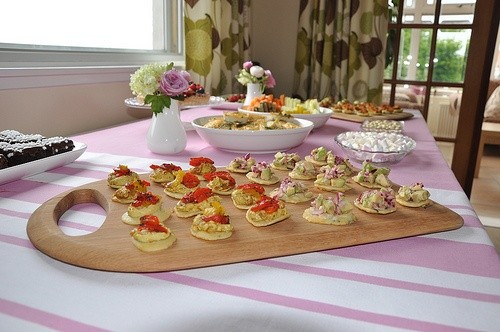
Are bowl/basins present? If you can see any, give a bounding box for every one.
[334,131,416,166]
[237,104,334,130]
[191,115,315,155]
[360,118,406,135]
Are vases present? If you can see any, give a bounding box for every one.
[242,83,261,105]
[146,98,186,155]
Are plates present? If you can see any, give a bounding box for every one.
[209,93,269,109]
[0,141,87,185]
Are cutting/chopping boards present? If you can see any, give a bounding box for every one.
[26,158,464,273]
[326,106,414,122]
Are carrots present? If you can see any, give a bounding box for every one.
[243,94,286,113]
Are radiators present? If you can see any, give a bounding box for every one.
[436,102,460,139]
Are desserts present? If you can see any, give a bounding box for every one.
[329,98,403,116]
[107,146,431,253]
[181,81,210,106]
[0,129,76,170]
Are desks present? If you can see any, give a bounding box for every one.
[0,106,500,332]
[473,121,500,178]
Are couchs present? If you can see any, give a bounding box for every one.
[383,86,424,109]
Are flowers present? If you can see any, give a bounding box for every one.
[234,60,276,93]
[128,61,191,118]
